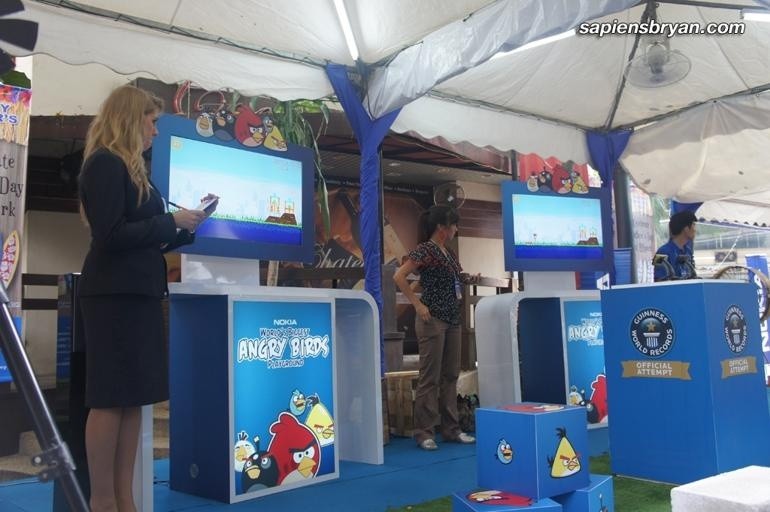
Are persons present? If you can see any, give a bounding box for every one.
[392,202,483,451]
[654,211,698,282]
[79,82,208,512]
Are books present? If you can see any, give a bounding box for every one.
[195,195,222,212]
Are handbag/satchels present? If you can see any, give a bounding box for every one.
[457,393,480,433]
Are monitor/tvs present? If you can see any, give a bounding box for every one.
[499,179,614,272]
[149,112,316,266]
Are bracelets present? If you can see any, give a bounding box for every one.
[189,229,196,234]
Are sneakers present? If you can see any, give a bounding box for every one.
[419,438,439,450]
[456,433,476,444]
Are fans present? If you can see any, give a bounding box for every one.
[618,3,693,91]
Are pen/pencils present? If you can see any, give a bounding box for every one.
[168,202,187,210]
